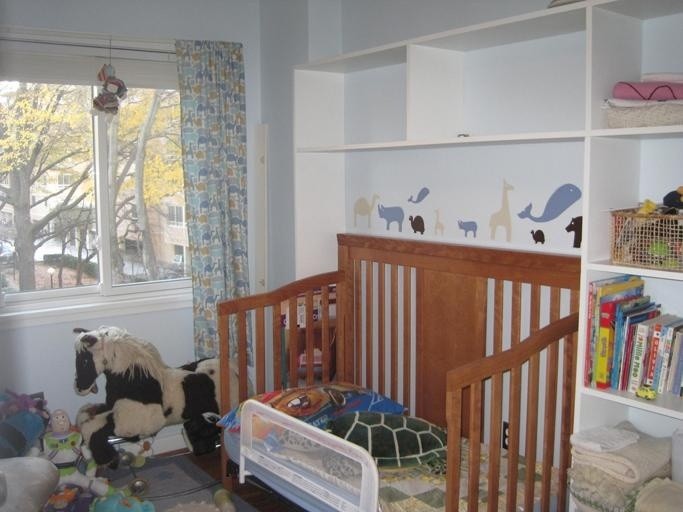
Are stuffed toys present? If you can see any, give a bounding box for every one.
[73,325,253,470]
[284,411,447,480]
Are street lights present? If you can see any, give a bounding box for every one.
[47,267,55,289]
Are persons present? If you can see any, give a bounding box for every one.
[26,410,147,496]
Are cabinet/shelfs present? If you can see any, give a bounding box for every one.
[292,2,681,512]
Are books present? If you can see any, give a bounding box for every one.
[585,276,683,399]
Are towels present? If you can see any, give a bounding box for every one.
[570,419,674,483]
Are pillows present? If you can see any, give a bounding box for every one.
[216,380,451,479]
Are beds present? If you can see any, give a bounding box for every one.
[214,233,581,512]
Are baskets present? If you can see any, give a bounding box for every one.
[608,203,683,275]
[602,104,683,128]
